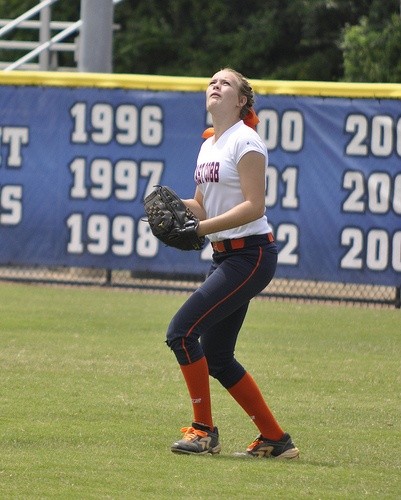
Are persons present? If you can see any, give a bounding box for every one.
[152,66,300,460]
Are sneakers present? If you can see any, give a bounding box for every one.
[170,421,221,455]
[233,432,300,461]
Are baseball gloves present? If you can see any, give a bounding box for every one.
[141,184,206,251]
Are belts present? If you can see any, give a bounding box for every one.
[210,232,274,252]
